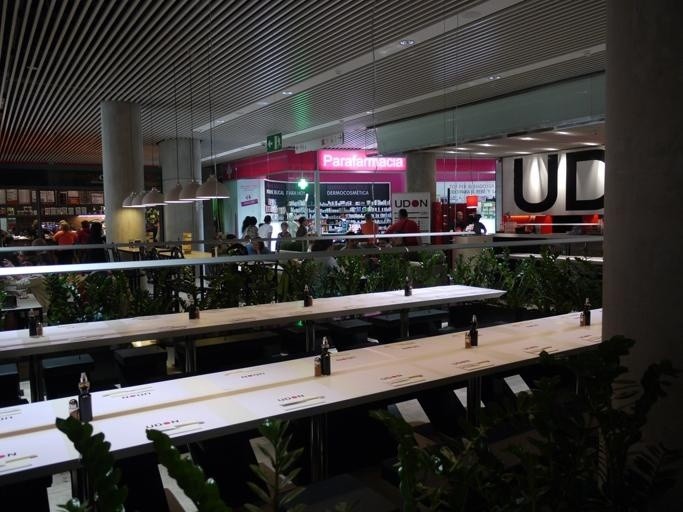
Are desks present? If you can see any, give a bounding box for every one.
[0,283,507,403]
[1,308,601,510]
[0,289,42,330]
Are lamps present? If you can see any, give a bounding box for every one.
[119,44,229,208]
[464,153,477,209]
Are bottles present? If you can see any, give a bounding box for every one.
[35,322,42,335]
[211,247,215,257]
[77,372,92,421]
[68,399,81,423]
[314,358,321,377]
[463,332,472,349]
[469,314,479,347]
[404,276,412,295]
[580,296,592,326]
[189,297,198,319]
[303,285,312,307]
[25,306,35,338]
[321,336,331,376]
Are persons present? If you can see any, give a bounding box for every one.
[146,219,160,248]
[226,209,487,298]
[0,220,105,329]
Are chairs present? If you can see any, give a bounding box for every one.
[97,232,259,308]
